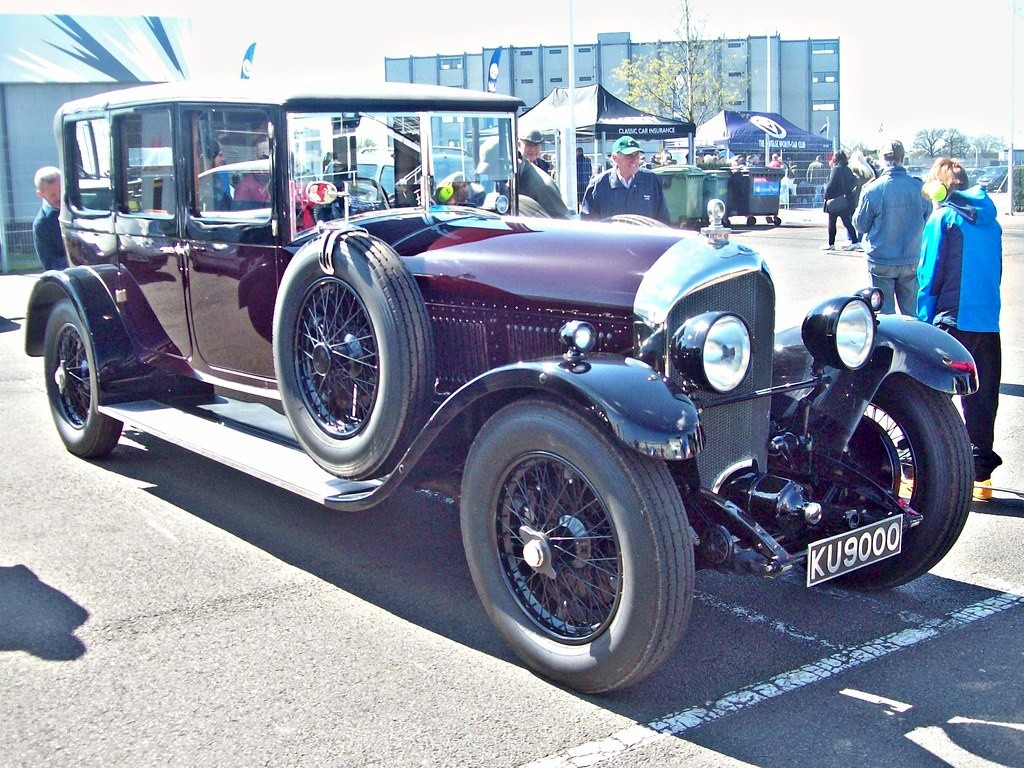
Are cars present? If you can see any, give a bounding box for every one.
[23,76,978,696]
[904,166,926,179]
[964,165,1008,193]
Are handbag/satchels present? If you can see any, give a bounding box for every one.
[826,194,850,213]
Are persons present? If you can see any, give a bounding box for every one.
[155,118,569,223]
[576,136,1002,500]
[32,167,70,270]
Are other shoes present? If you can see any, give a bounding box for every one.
[821,245,835,251]
[844,243,860,251]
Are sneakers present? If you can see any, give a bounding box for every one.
[972,481,993,500]
[898,478,915,499]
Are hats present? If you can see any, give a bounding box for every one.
[258,142,269,158]
[883,140,907,157]
[477,136,500,173]
[519,130,544,143]
[612,136,645,155]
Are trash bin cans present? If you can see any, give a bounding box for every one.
[702,168,731,227]
[720,166,786,226]
[650,164,708,231]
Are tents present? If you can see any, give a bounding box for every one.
[663,111,832,167]
[467,84,696,199]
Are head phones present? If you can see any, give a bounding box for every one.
[926,172,948,203]
[439,172,464,202]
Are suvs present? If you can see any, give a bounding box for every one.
[320,146,494,210]
